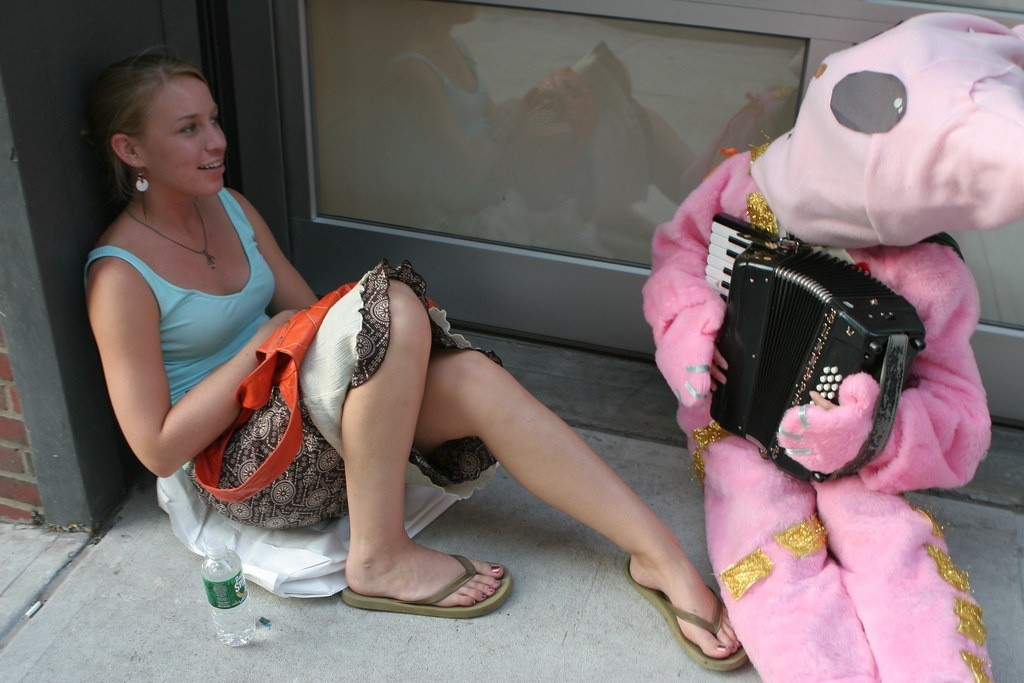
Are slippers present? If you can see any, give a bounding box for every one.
[625,554,748,672]
[340,554,513,618]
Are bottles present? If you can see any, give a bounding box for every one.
[201,539,256,648]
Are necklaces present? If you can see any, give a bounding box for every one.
[125,197,218,271]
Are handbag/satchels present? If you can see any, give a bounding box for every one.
[194,281,441,504]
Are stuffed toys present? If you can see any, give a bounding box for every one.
[639,9,1024,682]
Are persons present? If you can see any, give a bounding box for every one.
[77,52,749,676]
